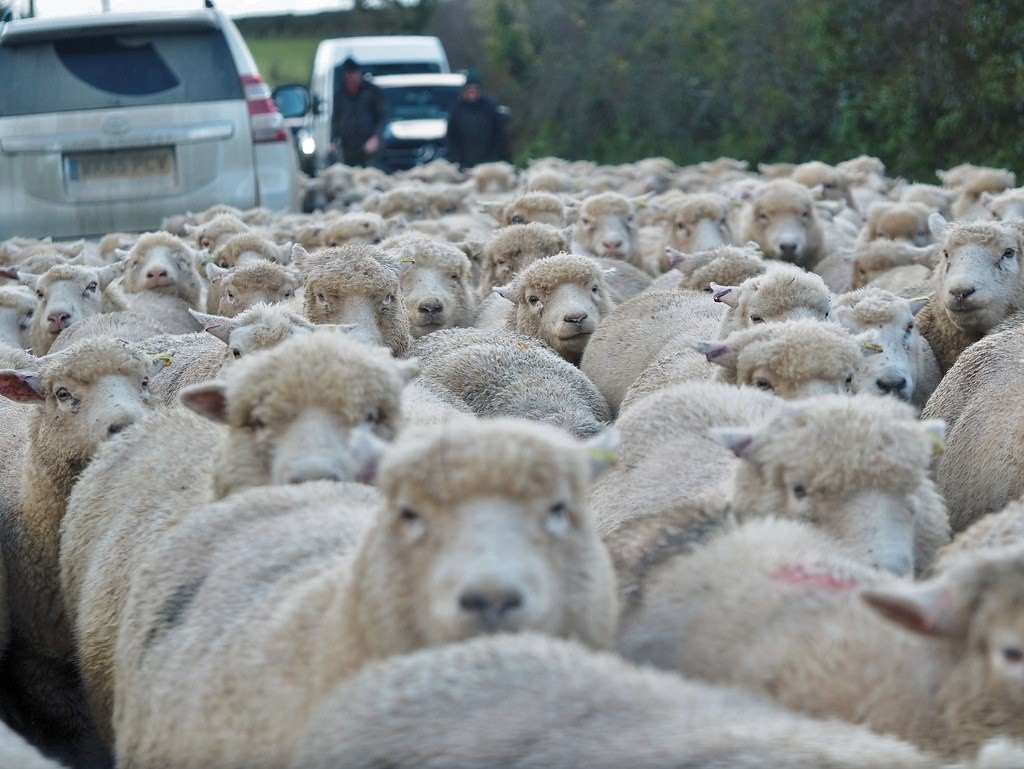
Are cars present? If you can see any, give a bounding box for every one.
[299,35,510,170]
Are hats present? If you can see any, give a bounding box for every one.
[466,71,482,86]
[342,60,359,72]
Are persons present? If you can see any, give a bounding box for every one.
[445,79,511,167]
[327,57,384,168]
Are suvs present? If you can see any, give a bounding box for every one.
[0,0,313,245]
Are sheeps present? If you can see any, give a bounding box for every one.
[583,320,1024,768]
[304,154,1024,356]
[1,205,623,769]
